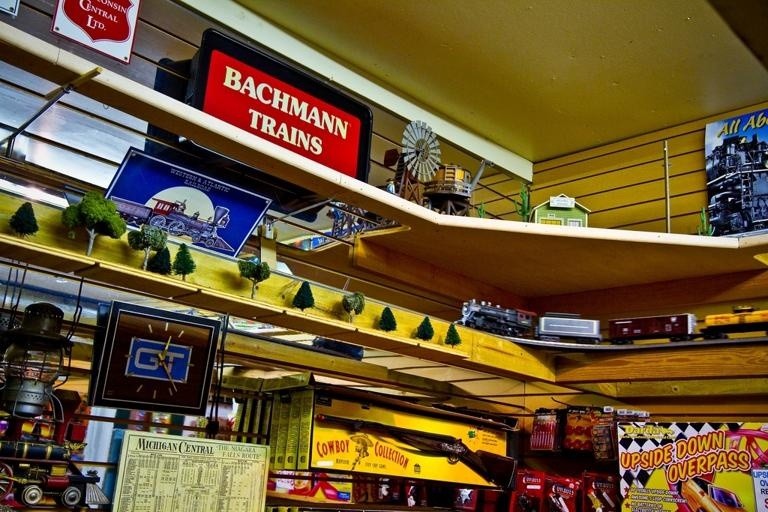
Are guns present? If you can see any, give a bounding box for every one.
[316,413,517,492]
